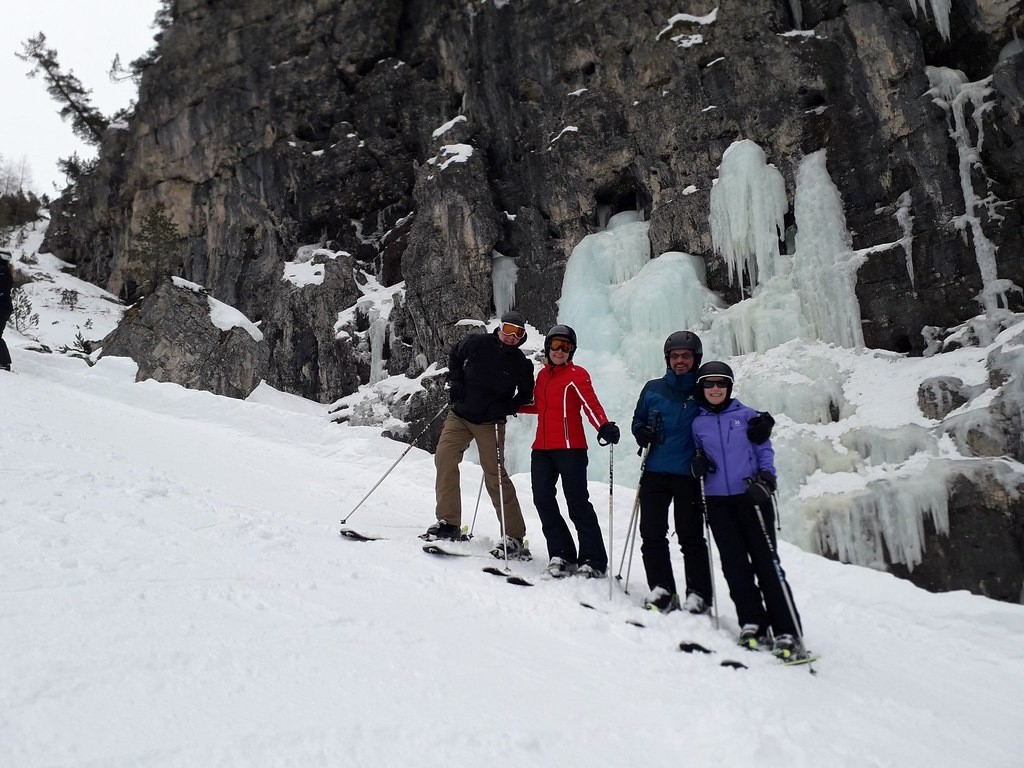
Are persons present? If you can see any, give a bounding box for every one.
[516,324,620,578]
[631,330,776,616]
[0,251,13,371]
[418,311,535,558]
[691,361,811,662]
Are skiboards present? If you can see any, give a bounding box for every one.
[679,639,816,669]
[338,529,469,558]
[579,600,646,628]
[483,566,535,587]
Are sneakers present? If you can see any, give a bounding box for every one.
[545,557,577,578]
[577,562,604,579]
[682,592,709,615]
[644,586,681,613]
[772,634,807,663]
[490,536,522,558]
[419,520,461,541]
[738,621,765,648]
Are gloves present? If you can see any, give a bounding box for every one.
[508,397,523,415]
[449,379,464,405]
[747,412,775,444]
[635,427,657,448]
[690,456,709,478]
[599,422,619,444]
[743,469,776,506]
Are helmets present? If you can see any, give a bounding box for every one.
[664,331,703,370]
[544,325,578,361]
[696,360,733,385]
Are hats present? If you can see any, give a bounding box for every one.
[501,311,524,327]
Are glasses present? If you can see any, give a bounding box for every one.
[668,352,694,359]
[702,379,730,388]
[501,322,525,340]
[550,339,575,352]
